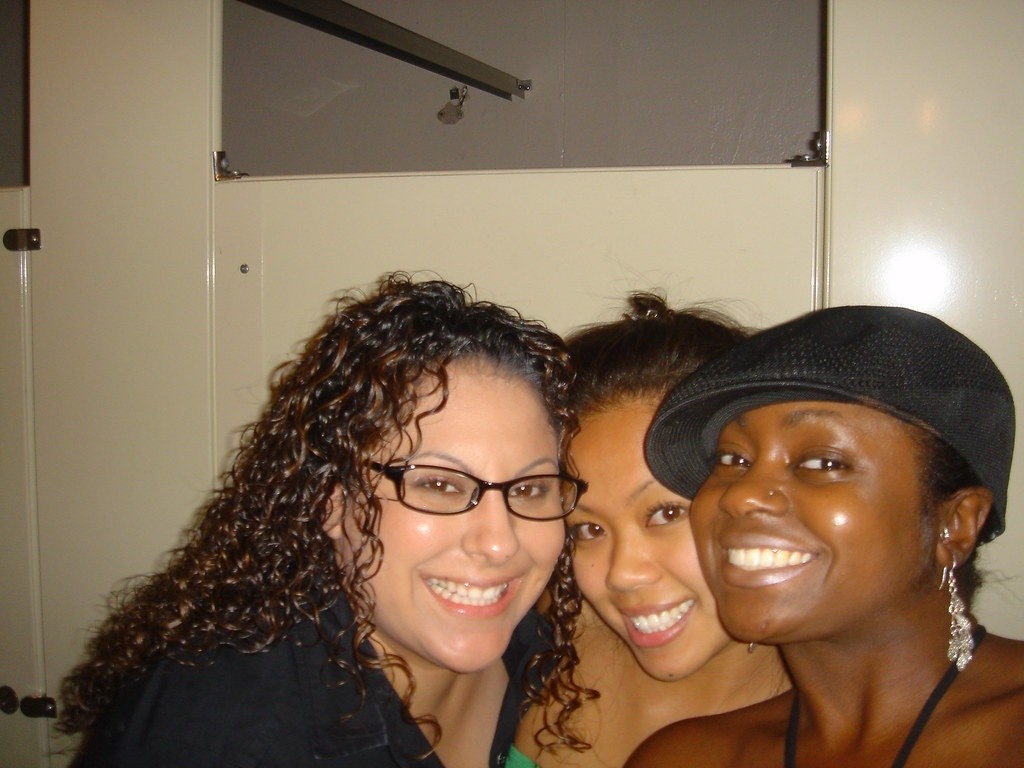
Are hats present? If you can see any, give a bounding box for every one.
[643,304,1017,550]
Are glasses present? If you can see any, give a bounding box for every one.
[371,457,588,523]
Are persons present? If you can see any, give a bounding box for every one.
[49,281,600,768]
[499,295,792,768]
[621,304,1023,768]
